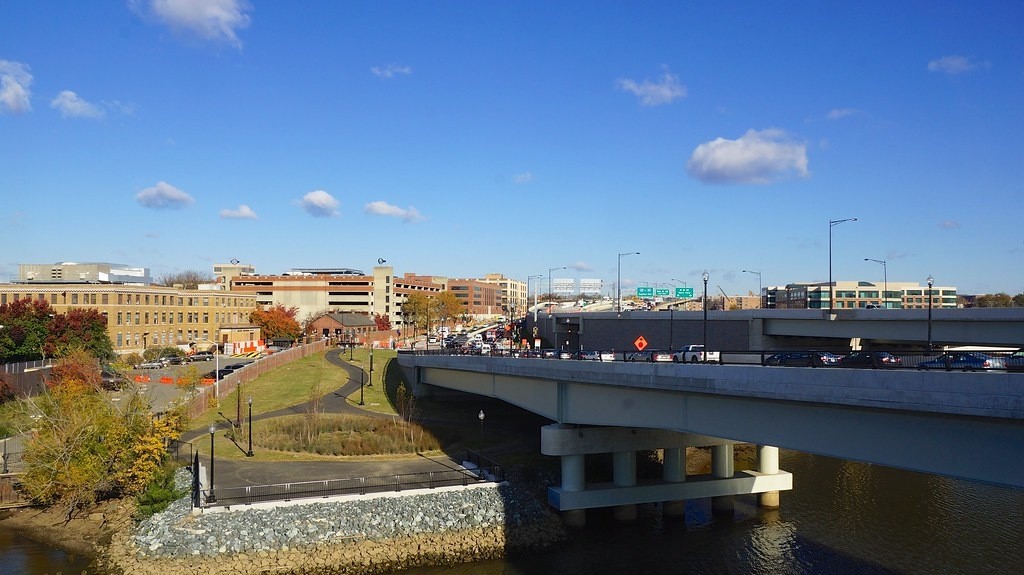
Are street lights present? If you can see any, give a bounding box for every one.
[205,421,217,504]
[865,257,888,309]
[196,337,220,407]
[368,353,373,386]
[440,316,444,349]
[547,266,567,315]
[742,269,762,310]
[829,218,858,309]
[245,396,255,456]
[509,303,515,358]
[617,252,640,312]
[540,276,551,303]
[672,278,687,310]
[343,331,346,354]
[350,335,353,360]
[662,282,677,309]
[527,274,542,312]
[701,270,709,364]
[668,303,674,354]
[358,365,366,406]
[925,274,935,356]
[477,409,486,482]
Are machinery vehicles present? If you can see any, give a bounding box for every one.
[717,286,741,310]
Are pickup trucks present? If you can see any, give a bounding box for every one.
[673,344,720,365]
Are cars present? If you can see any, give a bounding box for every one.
[779,348,840,369]
[835,350,902,371]
[93,350,254,394]
[762,352,788,367]
[1004,347,1024,372]
[866,304,887,309]
[628,347,673,363]
[915,349,1008,373]
[428,316,615,362]
